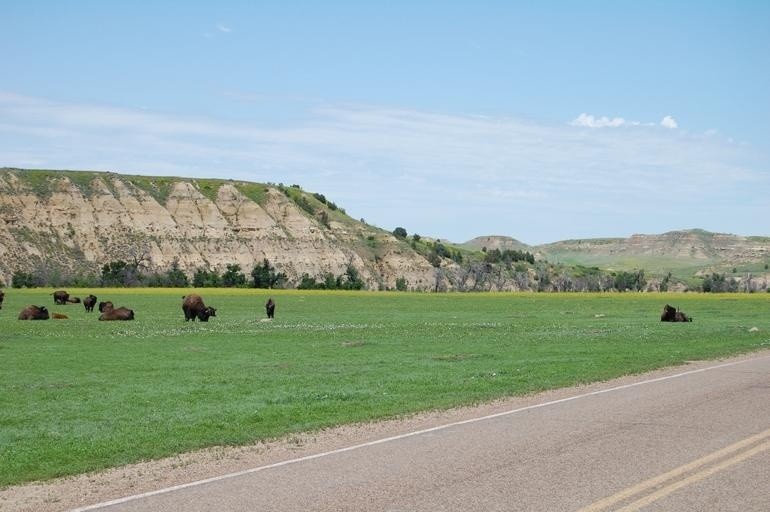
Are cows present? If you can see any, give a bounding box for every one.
[51,312,68,319]
[18,305,50,320]
[266,297,276,318]
[660,304,691,322]
[83,294,97,313]
[0,289,5,309]
[66,297,81,304]
[182,294,217,322]
[99,301,114,313]
[98,306,135,321]
[49,290,70,305]
